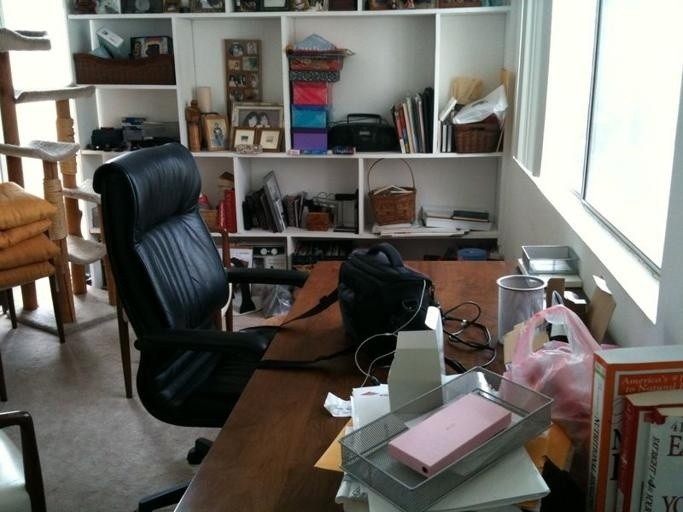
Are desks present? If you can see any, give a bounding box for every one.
[174,262,617,512]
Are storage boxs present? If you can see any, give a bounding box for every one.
[290,53,343,154]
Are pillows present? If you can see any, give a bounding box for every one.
[0,181,57,292]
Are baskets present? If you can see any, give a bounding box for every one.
[367,158,416,226]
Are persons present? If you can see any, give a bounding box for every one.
[213,122,224,147]
[230,40,272,129]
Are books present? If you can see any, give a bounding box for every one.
[335,374,551,512]
[392,86,457,154]
[584,344,683,512]
[370,206,493,238]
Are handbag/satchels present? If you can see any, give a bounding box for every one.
[339,242,435,365]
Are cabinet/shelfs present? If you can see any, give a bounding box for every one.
[66,4,509,288]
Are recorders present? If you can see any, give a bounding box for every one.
[328,113,401,152]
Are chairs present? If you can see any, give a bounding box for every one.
[92,142,315,510]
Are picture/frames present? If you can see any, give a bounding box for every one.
[232,126,257,152]
[204,116,230,151]
[229,102,283,153]
[224,37,262,123]
[256,127,283,152]
